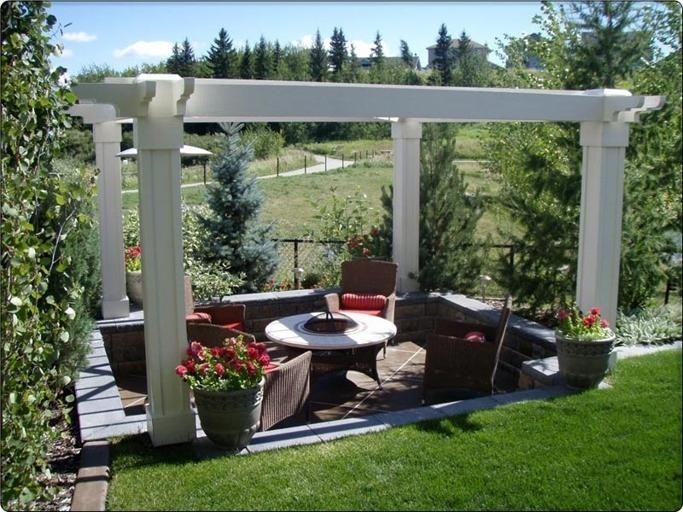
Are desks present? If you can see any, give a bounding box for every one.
[263,312,397,391]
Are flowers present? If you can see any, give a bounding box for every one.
[174,333,271,391]
[555,302,610,339]
[125,245,142,268]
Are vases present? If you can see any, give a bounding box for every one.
[193,376,265,450]
[126,270,142,308]
[554,328,616,392]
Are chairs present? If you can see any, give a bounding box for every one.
[324,260,399,345]
[420,294,512,403]
[184,274,313,431]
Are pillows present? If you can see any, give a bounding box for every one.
[341,290,387,310]
[464,331,485,343]
